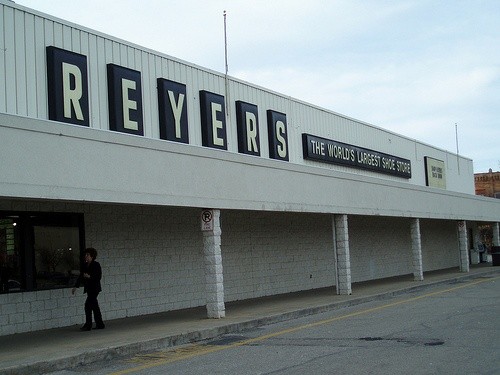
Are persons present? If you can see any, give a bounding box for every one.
[72,246,105,331]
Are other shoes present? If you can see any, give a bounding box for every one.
[92,325,105,329]
[80,325,91,331]
